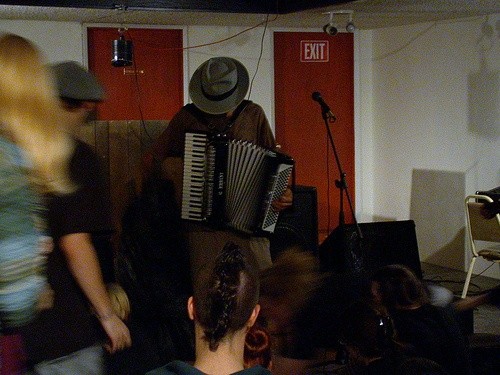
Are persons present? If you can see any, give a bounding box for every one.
[33,61,131,375]
[0,33,75,375]
[147,242,272,375]
[372,264,474,375]
[243,324,272,370]
[341,303,440,375]
[145,58,292,271]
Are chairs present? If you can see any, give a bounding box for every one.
[461,194,500,299]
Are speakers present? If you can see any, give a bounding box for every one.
[319,219,424,285]
[120,203,191,295]
[262,185,318,265]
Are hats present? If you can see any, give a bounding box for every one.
[54,62,104,100]
[188,56,250,114]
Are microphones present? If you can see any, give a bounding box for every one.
[311,92,335,119]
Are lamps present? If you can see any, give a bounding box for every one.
[323,9,357,38]
